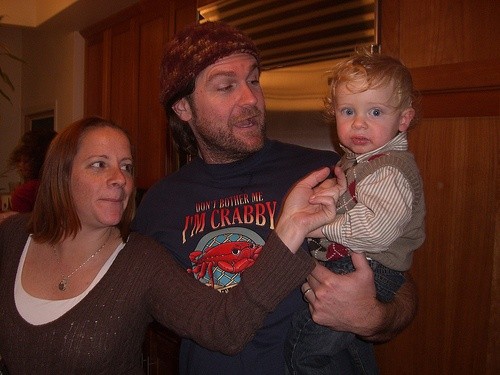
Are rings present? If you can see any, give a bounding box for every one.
[303,288,312,303]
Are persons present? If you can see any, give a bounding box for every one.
[0,117,347,375]
[129,21,417,375]
[281,54,425,375]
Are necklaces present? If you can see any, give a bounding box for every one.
[51,227,113,291]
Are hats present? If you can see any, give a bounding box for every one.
[158,20,262,105]
